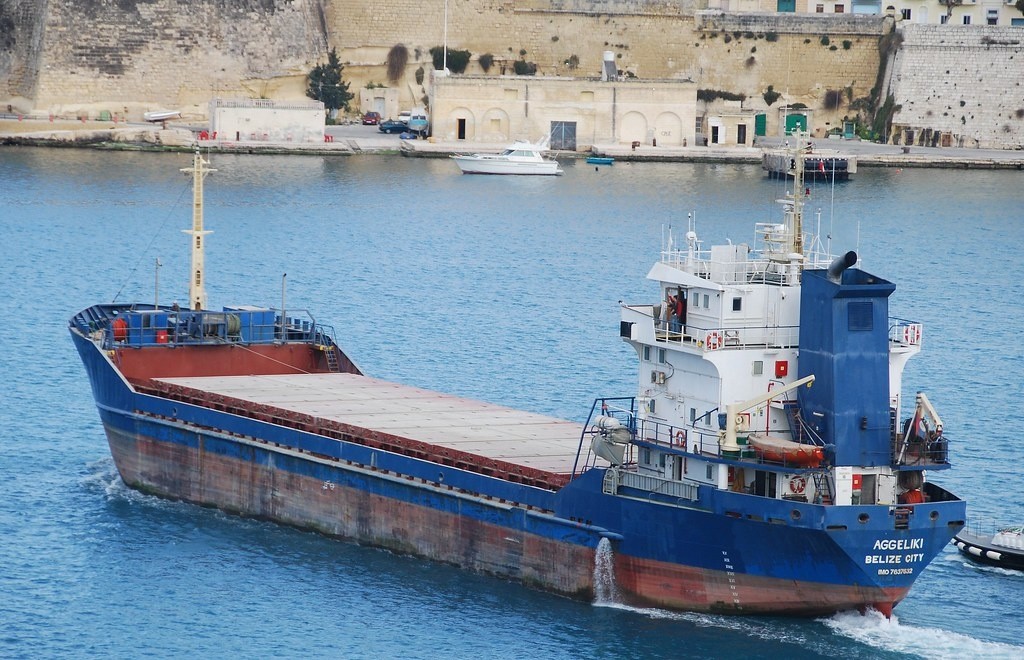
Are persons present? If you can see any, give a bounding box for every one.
[667,286,684,340]
[901,482,925,511]
[172,300,180,312]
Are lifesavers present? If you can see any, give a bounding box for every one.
[675,430,686,448]
[790,473,805,495]
[904,325,920,344]
[706,332,722,350]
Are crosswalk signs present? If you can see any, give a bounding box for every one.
[67,84,972,624]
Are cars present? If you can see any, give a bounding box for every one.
[378,120,409,134]
[397,110,411,124]
[362,111,381,125]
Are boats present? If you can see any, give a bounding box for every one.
[747,430,836,468]
[949,516,1024,571]
[142,108,182,121]
[585,153,616,165]
[760,120,858,182]
[447,120,566,175]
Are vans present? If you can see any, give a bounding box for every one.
[409,109,427,120]
